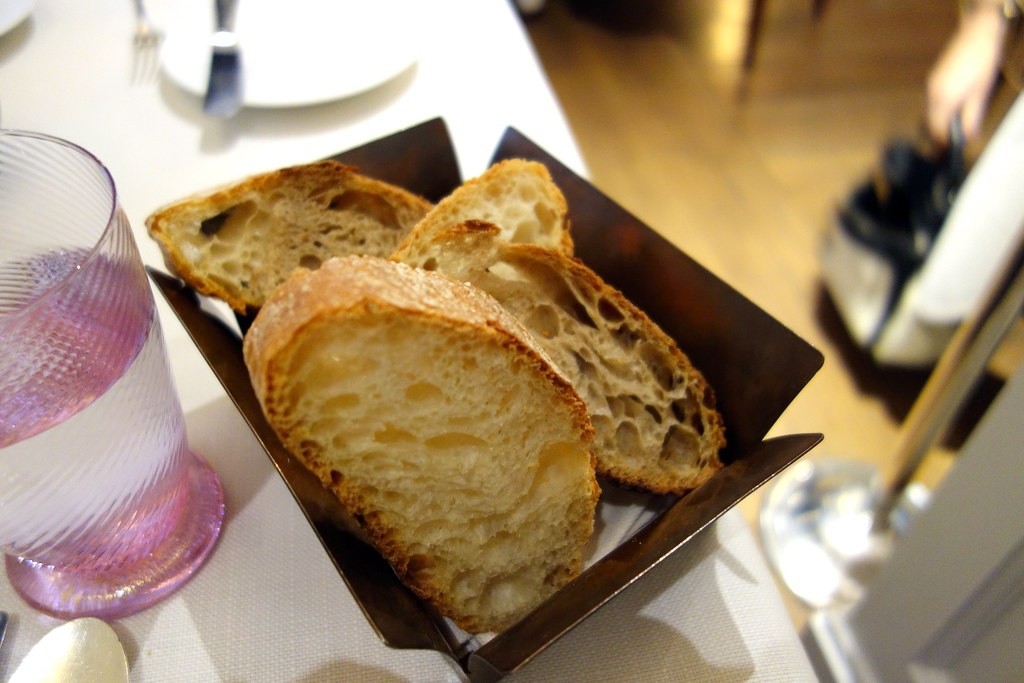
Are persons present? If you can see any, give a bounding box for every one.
[925,0,1024,143]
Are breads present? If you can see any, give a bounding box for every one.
[144,157,437,316]
[390,155,573,264]
[242,255,602,636]
[409,221,727,495]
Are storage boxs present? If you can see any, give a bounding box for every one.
[141,118,827,683]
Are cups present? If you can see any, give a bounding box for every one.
[0,130,224,623]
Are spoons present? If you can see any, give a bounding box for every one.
[5,617,129,683]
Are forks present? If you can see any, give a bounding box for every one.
[128,0,159,79]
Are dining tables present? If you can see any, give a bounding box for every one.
[0,0,821,683]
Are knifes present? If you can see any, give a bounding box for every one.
[202,0,243,117]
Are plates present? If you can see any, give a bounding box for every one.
[158,0,420,110]
[0,0,36,37]
